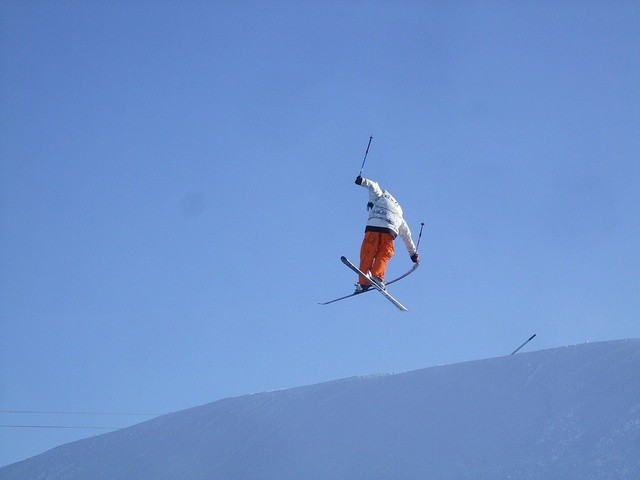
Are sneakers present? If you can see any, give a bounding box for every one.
[354,284,372,293]
[372,275,385,289]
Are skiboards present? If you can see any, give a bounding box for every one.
[316,256,420,312]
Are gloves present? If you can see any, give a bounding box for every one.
[355,176,363,185]
[411,254,419,262]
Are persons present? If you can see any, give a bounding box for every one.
[354,175,419,290]
